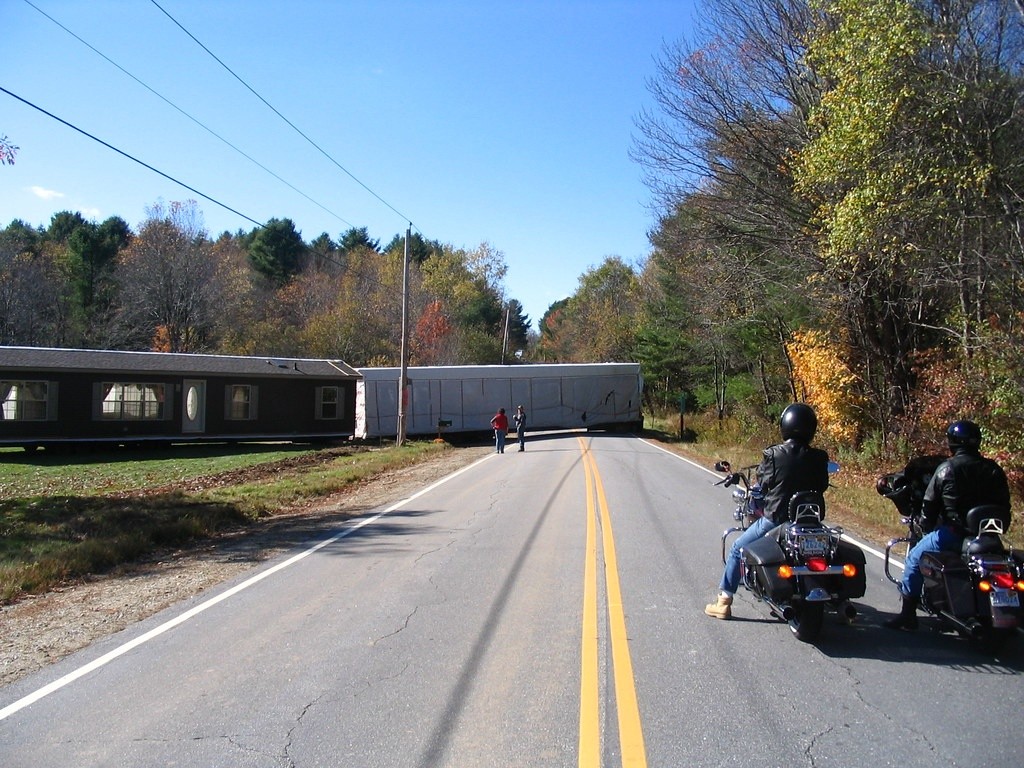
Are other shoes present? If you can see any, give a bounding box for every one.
[497,451,499,453]
[501,449,504,453]
[518,446,524,452]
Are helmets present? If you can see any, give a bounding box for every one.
[944,420,982,453]
[779,403,818,445]
[719,461,726,467]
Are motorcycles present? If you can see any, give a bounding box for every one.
[876,455,1024,655]
[712,462,867,641]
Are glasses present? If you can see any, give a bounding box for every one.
[723,462,729,466]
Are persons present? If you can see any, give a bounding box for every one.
[513,404,527,452]
[879,477,891,494]
[704,402,857,625]
[718,461,730,473]
[490,407,508,453]
[882,419,1010,631]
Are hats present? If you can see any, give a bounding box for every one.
[518,406,523,409]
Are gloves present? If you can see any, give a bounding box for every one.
[915,514,922,523]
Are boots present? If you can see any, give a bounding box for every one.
[705,594,733,619]
[836,599,855,625]
[882,597,919,632]
